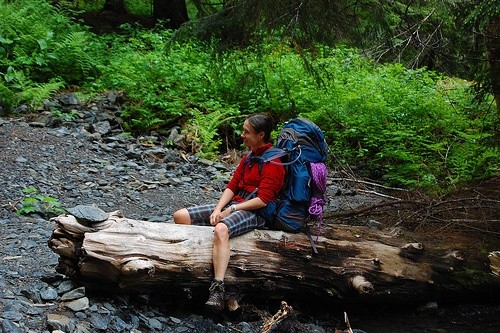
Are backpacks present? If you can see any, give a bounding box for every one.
[257,118,329,234]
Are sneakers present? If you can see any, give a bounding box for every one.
[205,279,226,308]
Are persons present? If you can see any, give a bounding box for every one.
[174,112,285,308]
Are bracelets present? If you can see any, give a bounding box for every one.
[231,204,236,211]
